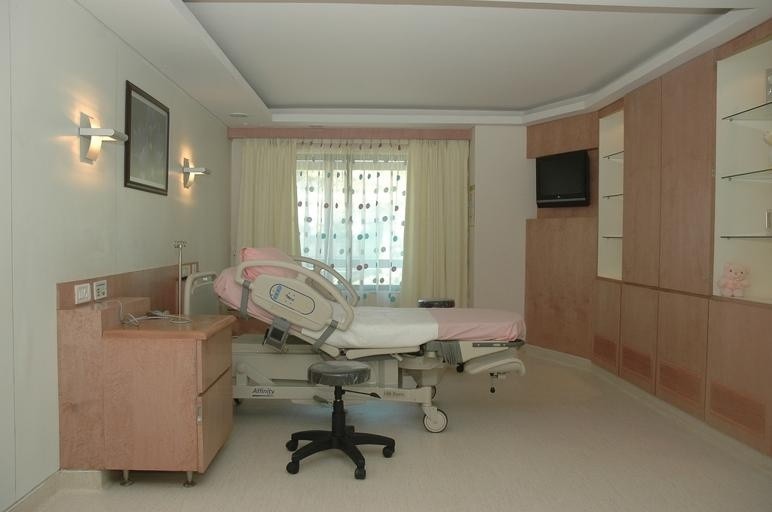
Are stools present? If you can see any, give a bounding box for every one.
[285,357,397,479]
[415,298,455,309]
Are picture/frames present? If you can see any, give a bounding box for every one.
[125,80,170,197]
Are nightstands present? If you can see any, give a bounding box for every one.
[104,312,236,486]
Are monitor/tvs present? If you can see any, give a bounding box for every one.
[536,150,590,208]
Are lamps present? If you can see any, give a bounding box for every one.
[182,157,213,190]
[77,113,128,164]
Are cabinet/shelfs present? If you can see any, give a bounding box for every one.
[719,99,772,240]
[592,279,659,399]
[622,51,717,296]
[598,147,624,240]
[652,289,771,463]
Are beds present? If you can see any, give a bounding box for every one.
[183,249,528,432]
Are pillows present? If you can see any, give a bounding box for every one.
[240,244,298,279]
